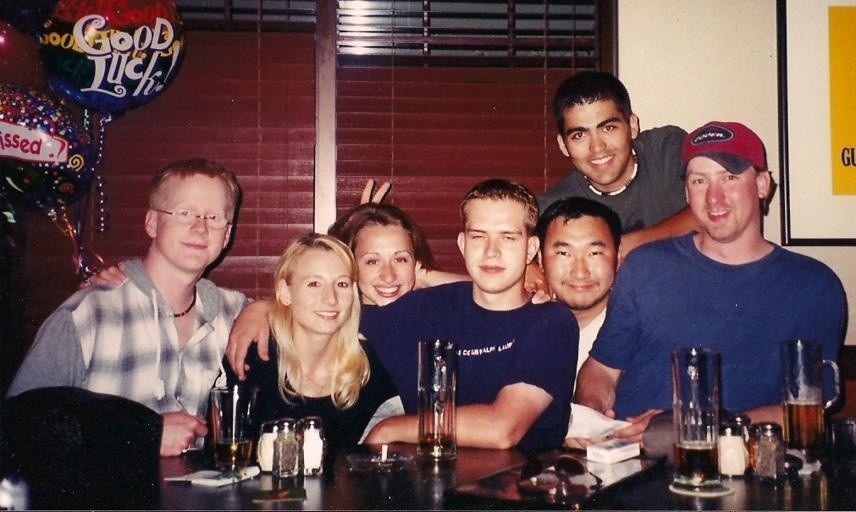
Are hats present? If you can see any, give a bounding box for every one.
[679,121,766,177]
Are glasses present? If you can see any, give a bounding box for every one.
[151,207,232,229]
[521,458,602,488]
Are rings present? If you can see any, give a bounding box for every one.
[182,444,192,453]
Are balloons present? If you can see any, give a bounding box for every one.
[36,0,187,121]
[0,75,93,210]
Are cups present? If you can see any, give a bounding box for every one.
[419,339,459,460]
[672,346,720,485]
[213,388,253,474]
[780,340,836,461]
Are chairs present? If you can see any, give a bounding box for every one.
[0,386,166,512]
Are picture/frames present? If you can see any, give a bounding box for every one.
[776,0,856,247]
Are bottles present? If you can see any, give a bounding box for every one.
[750,423,784,481]
[257,420,275,473]
[272,417,301,478]
[301,417,327,476]
[717,417,748,480]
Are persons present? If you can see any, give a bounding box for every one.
[522,70,707,305]
[562,120,848,450]
[531,195,624,404]
[206,232,406,455]
[227,177,579,452]
[323,203,468,306]
[0,23,44,88]
[5,157,390,460]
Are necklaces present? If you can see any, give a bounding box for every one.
[584,149,638,198]
[174,285,196,317]
